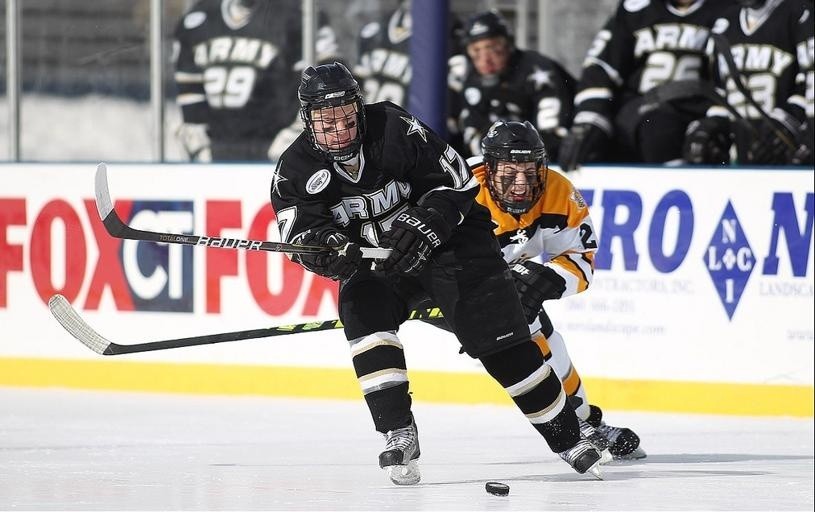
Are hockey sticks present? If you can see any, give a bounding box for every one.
[93,162,393,259]
[48,294,446,355]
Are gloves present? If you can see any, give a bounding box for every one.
[302,227,363,281]
[559,124,597,172]
[378,208,448,275]
[748,117,801,163]
[510,260,566,323]
[684,117,726,163]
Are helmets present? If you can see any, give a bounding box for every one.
[482,120,547,214]
[298,62,366,161]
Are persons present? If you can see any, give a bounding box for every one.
[685,0,814,168]
[556,0,730,172]
[271,61,604,475]
[350,0,460,110]
[469,119,640,456]
[174,0,342,165]
[448,11,580,159]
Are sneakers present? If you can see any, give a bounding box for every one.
[558,416,639,474]
[379,416,420,468]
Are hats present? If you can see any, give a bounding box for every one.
[460,12,506,45]
